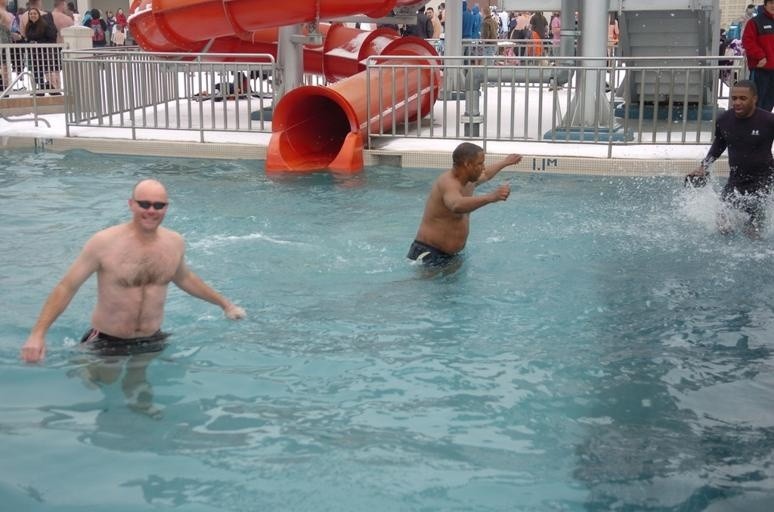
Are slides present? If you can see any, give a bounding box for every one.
[125,0,443,176]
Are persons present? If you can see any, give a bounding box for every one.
[235,73,251,93]
[403,0,620,73]
[20,177,247,420]
[1,0,130,98]
[214,80,239,95]
[683,77,774,239]
[403,141,524,264]
[718,0,774,112]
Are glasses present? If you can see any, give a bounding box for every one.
[134,200,167,210]
